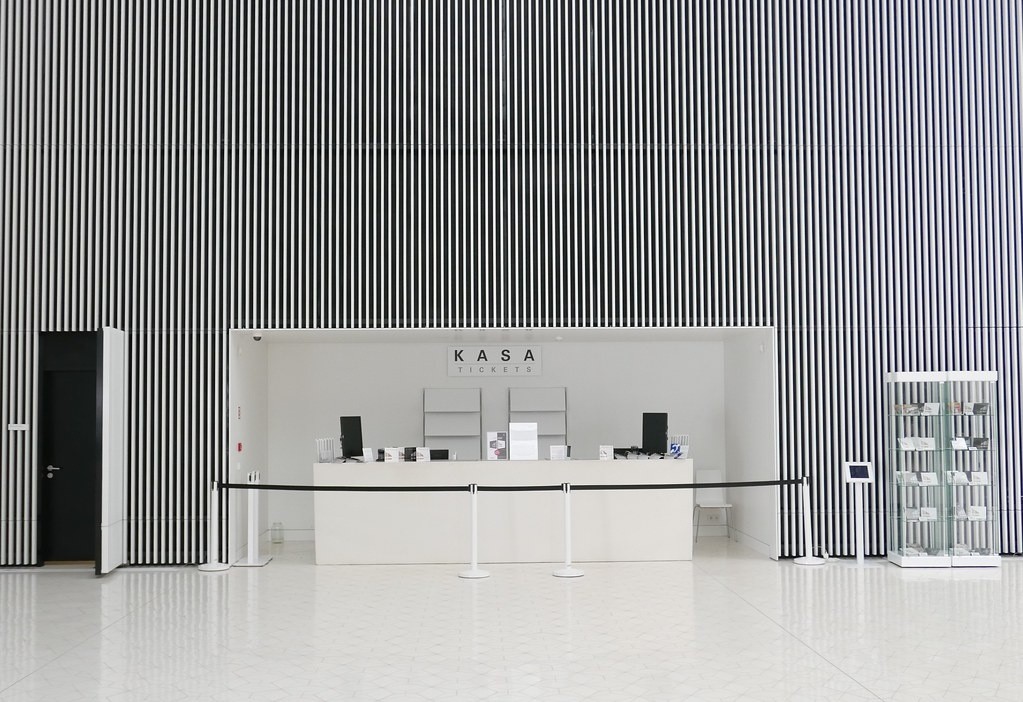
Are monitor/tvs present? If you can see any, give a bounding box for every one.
[340,416,364,458]
[643,413,667,453]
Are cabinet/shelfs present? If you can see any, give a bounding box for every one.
[945,371,1002,567]
[509,386,567,460]
[885,371,950,567]
[423,388,481,460]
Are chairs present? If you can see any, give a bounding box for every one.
[693,470,736,543]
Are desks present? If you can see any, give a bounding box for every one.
[314,458,693,566]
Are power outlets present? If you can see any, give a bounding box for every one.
[707,514,720,521]
